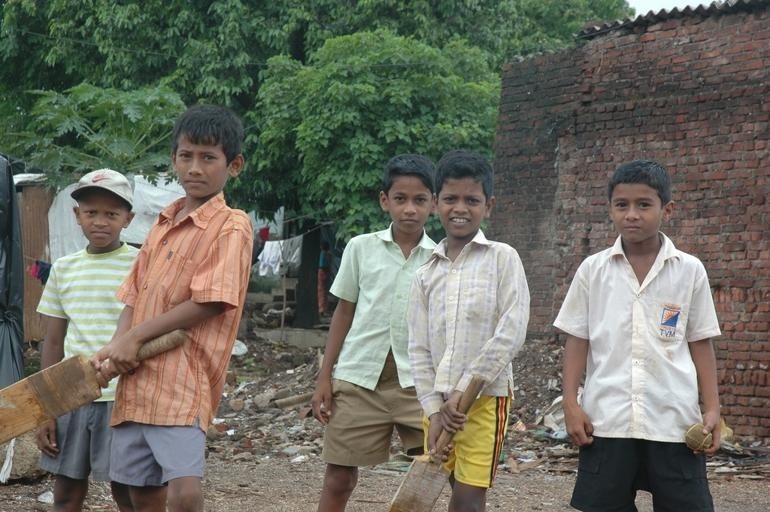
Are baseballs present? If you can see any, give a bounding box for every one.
[685,423,713,452]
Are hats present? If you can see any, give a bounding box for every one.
[71,168,134,207]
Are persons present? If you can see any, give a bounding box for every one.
[32,168,143,512]
[312,152,440,512]
[552,158,724,511]
[317,240,333,316]
[89,102,255,511]
[406,150,533,511]
